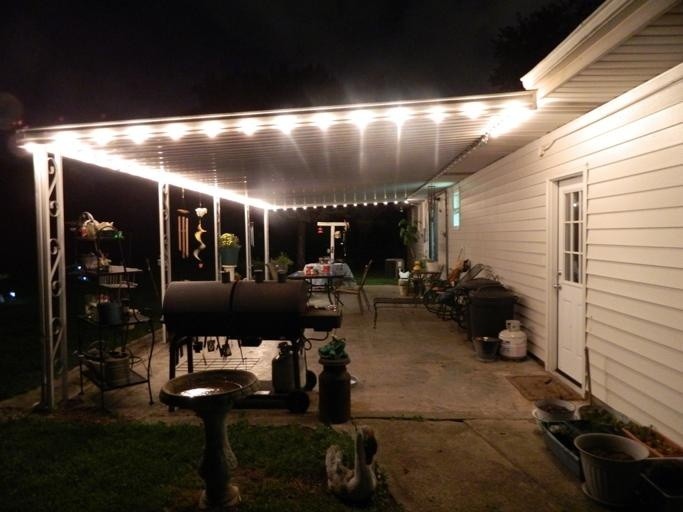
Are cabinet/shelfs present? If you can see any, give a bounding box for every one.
[71,211,164,414]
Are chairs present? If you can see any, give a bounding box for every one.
[422,262,483,329]
[333,259,372,314]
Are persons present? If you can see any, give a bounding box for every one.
[417,259,472,300]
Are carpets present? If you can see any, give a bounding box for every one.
[505,371,584,403]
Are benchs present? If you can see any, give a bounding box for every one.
[371,297,435,329]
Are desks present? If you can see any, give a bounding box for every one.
[410,268,441,307]
[288,270,346,308]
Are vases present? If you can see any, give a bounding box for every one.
[531,395,682,512]
[473,336,503,362]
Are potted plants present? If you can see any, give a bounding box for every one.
[269,252,293,274]
[219,233,241,265]
